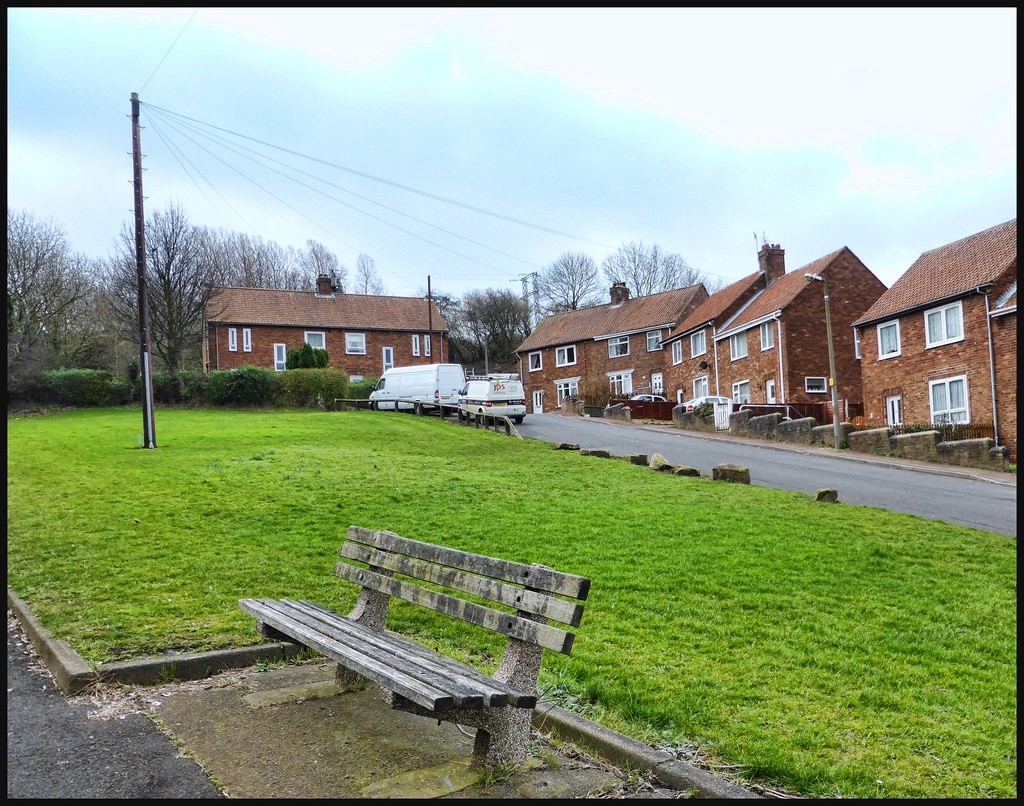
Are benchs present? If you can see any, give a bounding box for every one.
[237,524,590,776]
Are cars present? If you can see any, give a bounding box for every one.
[677,395,739,415]
[604,395,668,408]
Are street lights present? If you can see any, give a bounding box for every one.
[804,270,840,448]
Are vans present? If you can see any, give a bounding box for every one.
[367,363,467,417]
[457,377,526,425]
